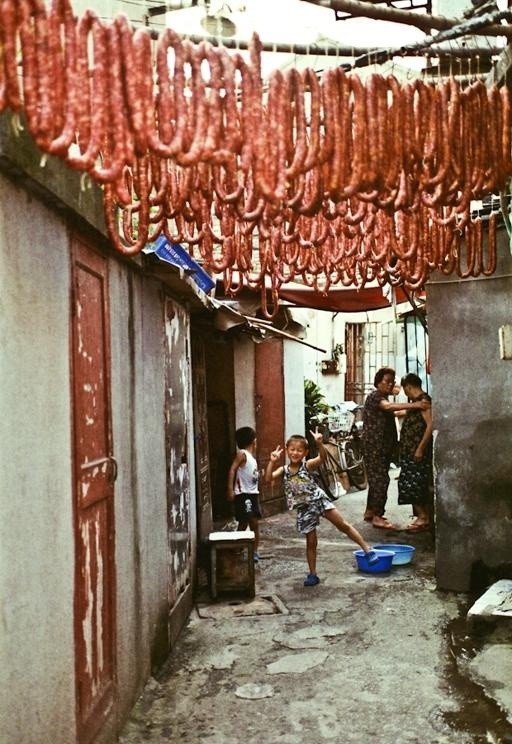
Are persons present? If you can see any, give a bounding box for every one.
[264,424,380,586]
[387,380,410,479]
[226,426,265,561]
[362,366,432,529]
[398,372,435,533]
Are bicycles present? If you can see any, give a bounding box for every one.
[306,403,368,501]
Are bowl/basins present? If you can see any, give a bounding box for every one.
[371,544,415,565]
[353,549,396,572]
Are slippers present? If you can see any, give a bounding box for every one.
[373,520,396,530]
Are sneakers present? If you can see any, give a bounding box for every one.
[404,523,431,533]
[253,551,259,562]
[363,513,387,521]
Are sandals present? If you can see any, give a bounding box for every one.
[364,547,380,567]
[304,573,320,587]
